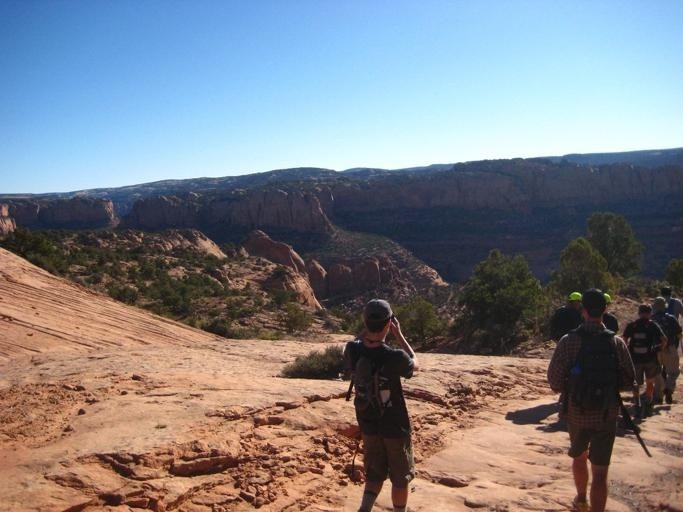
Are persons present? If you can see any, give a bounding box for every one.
[549,284,683,419]
[546,288,636,512]
[342,298,420,512]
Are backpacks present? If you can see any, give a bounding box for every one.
[628,319,655,357]
[346,350,399,423]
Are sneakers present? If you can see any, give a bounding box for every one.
[573,495,588,512]
[634,387,673,413]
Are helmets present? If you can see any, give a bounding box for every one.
[568,292,582,301]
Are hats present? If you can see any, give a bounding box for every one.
[654,297,664,304]
[364,299,392,323]
[603,293,612,304]
[565,331,614,411]
[582,288,605,307]
[638,304,652,313]
